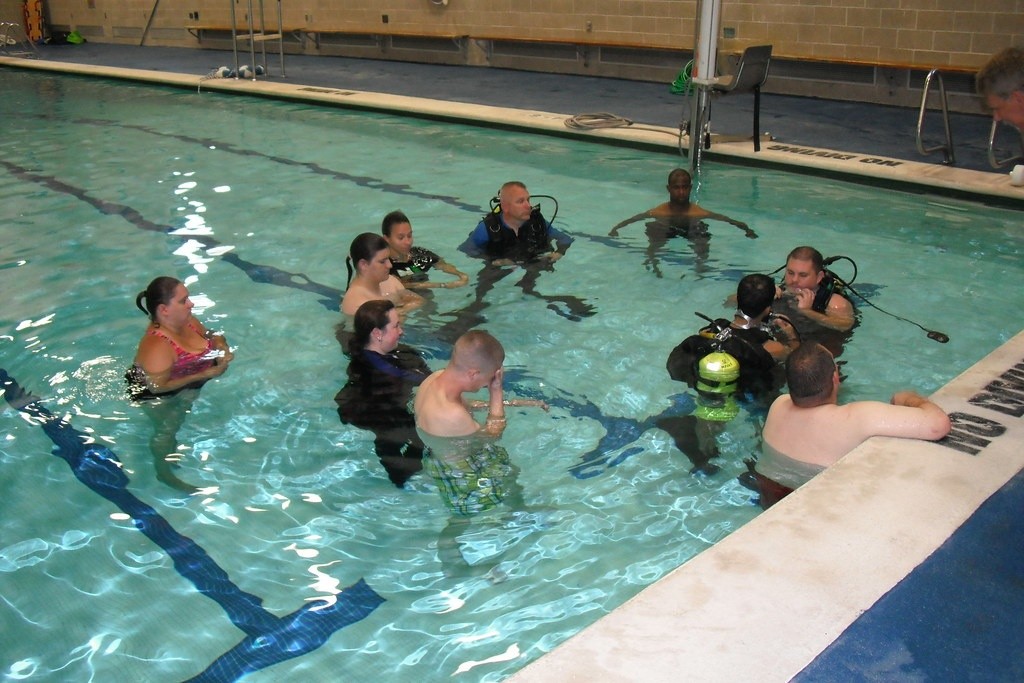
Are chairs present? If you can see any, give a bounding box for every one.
[704,43,772,152]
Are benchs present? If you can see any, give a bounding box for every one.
[185,23,985,77]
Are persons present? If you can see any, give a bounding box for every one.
[647,168,712,215]
[461,181,570,251]
[414,329,504,438]
[381,211,441,278]
[342,232,405,315]
[975,48,1024,187]
[133,278,229,372]
[700,274,801,372]
[773,246,853,313]
[348,300,403,358]
[762,340,950,468]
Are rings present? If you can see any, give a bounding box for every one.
[799,290,801,292]
